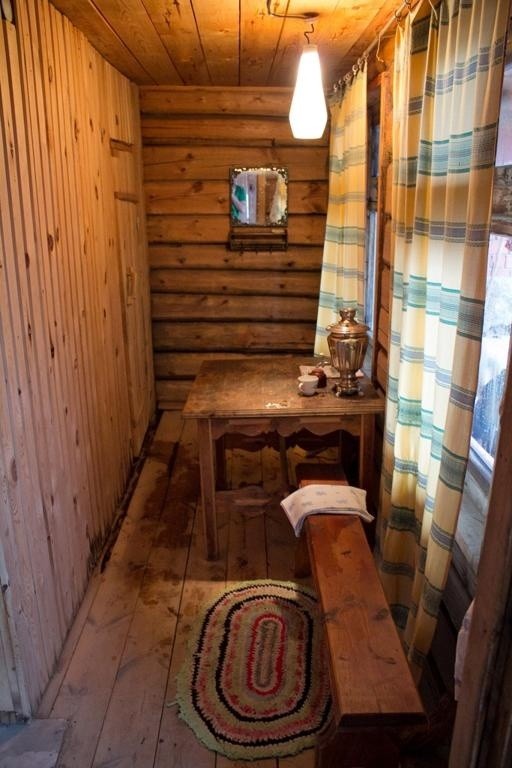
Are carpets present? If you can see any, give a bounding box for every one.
[168,575,333,761]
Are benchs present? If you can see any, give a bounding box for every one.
[291,458,430,768]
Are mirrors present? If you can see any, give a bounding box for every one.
[227,162,288,228]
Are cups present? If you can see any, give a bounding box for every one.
[298,375,319,396]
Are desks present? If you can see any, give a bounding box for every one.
[182,356,386,562]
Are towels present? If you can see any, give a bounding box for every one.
[279,483,376,536]
